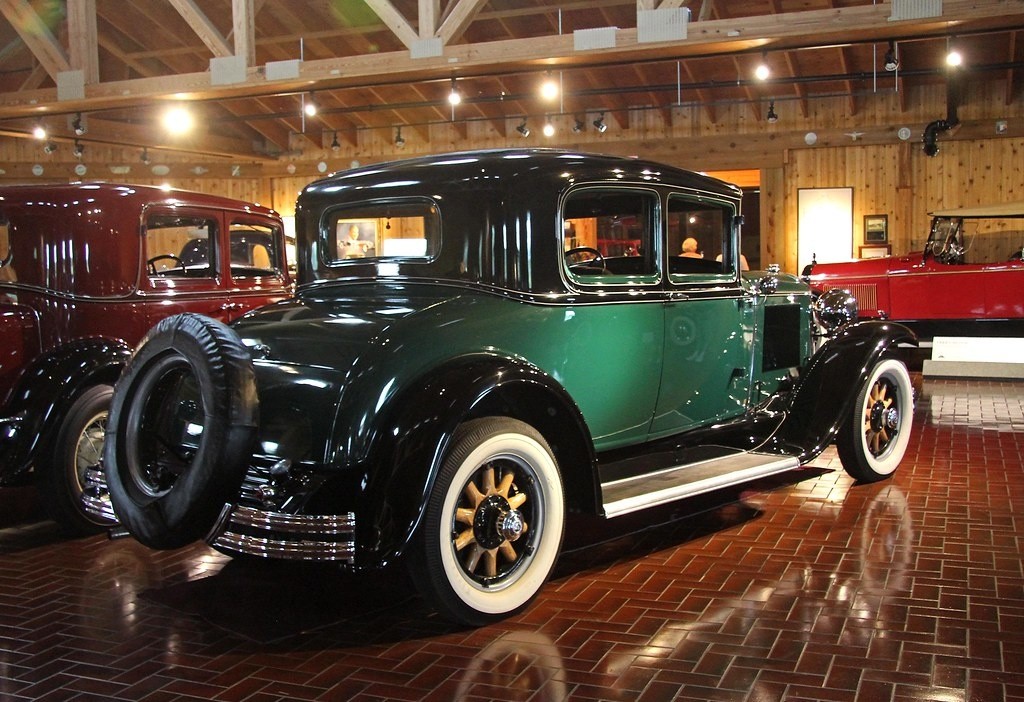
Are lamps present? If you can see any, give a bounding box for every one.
[767,100,779,124]
[44,114,86,157]
[572,111,607,133]
[884,40,900,71]
[515,117,530,137]
[330,132,341,152]
[395,126,405,146]
[140,147,152,165]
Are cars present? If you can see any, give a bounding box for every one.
[800,201,1024,319]
[103,150,918,617]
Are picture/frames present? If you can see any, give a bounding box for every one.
[337,219,377,261]
[858,214,891,260]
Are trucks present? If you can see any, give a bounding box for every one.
[0,184,295,533]
